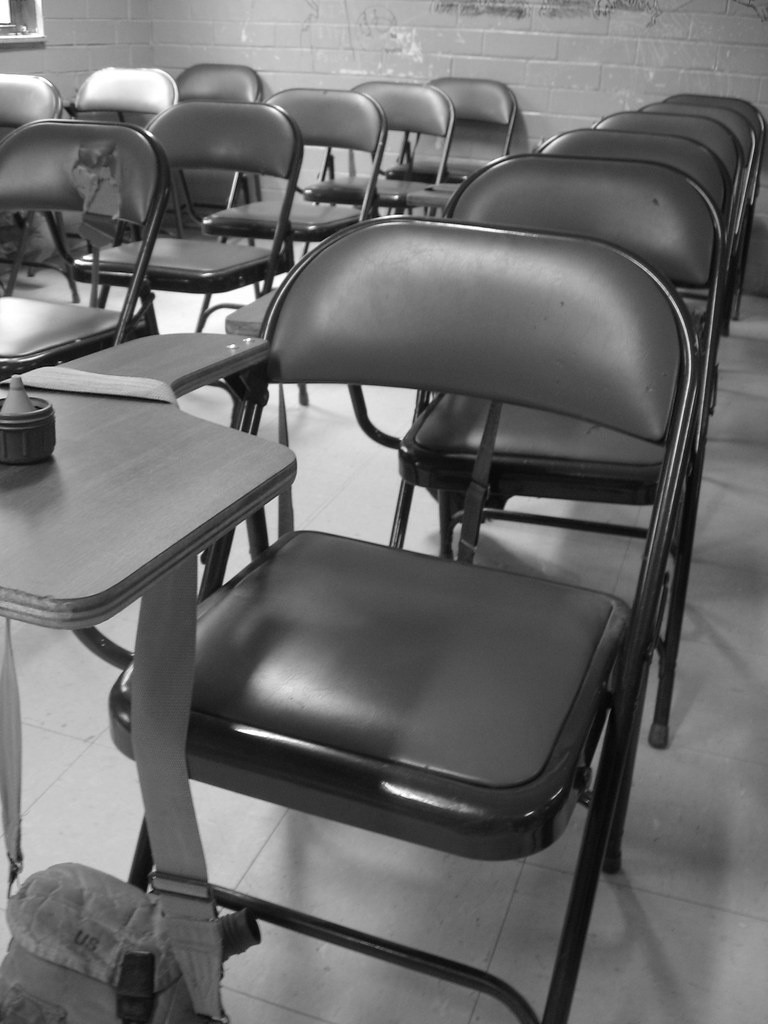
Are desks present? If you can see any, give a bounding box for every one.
[0,334,296,629]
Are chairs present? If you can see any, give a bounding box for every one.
[0,73,80,305]
[534,92,765,336]
[304,80,454,256]
[70,102,304,333]
[197,87,388,335]
[159,64,262,238]
[386,75,518,216]
[0,119,170,382]
[74,66,184,309]
[111,213,696,1024]
[390,153,723,752]
